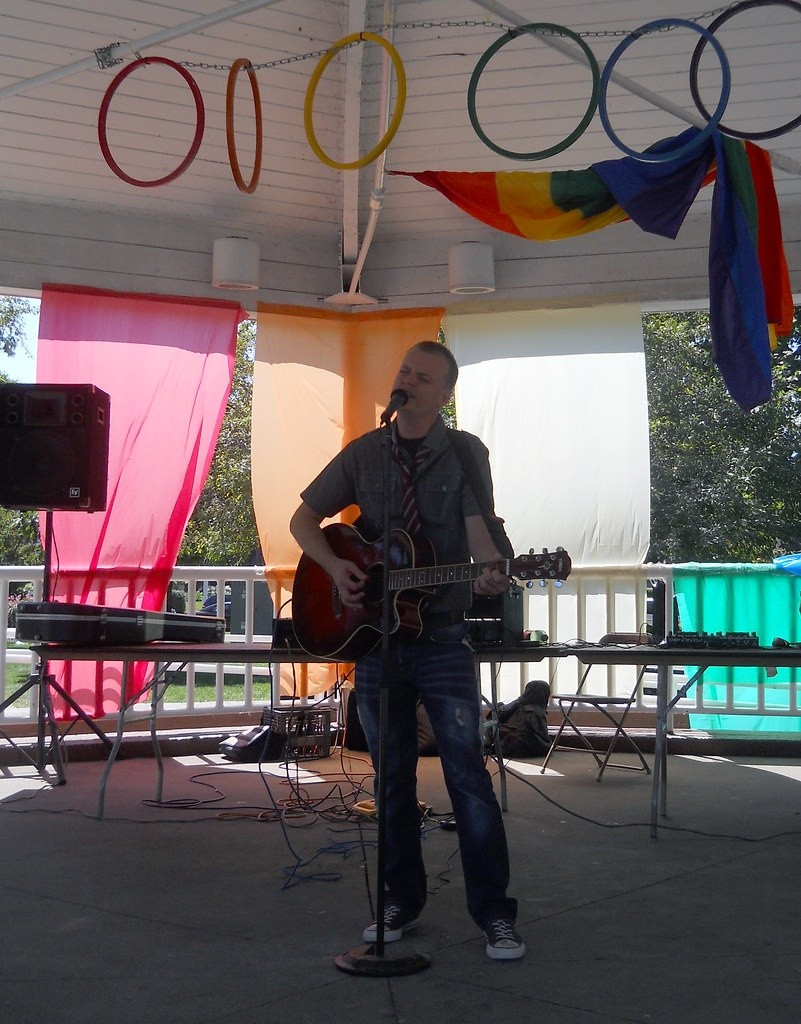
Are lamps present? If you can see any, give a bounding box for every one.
[212,236,261,290]
[448,241,495,295]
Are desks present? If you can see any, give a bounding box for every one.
[31,640,561,818]
[566,646,801,838]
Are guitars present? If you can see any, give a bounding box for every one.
[290,522,573,663]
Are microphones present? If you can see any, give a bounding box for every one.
[379,389,408,422]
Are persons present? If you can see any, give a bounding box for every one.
[290,341,525,959]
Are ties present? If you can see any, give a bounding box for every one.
[390,433,433,613]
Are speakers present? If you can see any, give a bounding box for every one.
[0,383,111,513]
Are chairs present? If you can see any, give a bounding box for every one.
[541,633,653,783]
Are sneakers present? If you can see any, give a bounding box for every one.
[484,916,526,960]
[363,904,420,943]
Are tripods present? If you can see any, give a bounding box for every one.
[0,508,124,785]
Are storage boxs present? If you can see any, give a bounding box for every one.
[263,706,330,762]
